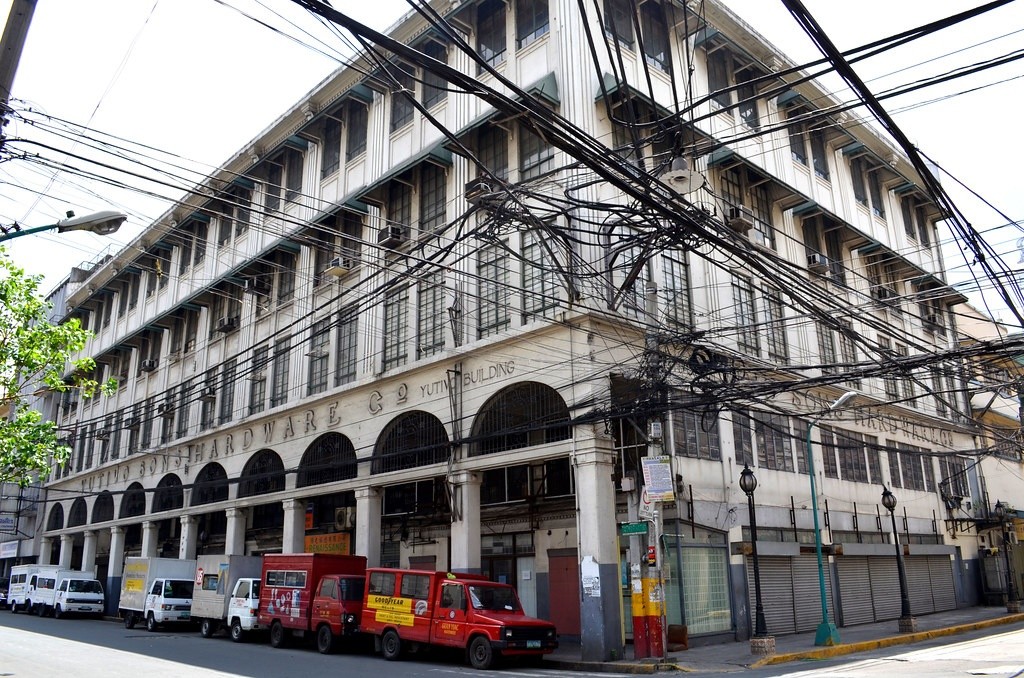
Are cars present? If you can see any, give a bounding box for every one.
[0,577,10,609]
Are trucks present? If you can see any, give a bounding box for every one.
[32,568,104,619]
[118,556,197,632]
[361,567,557,669]
[190,554,264,643]
[7,564,65,614]
[258,553,367,655]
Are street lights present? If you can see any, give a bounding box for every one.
[994,498,1018,601]
[806,391,859,645]
[881,485,916,619]
[738,462,774,639]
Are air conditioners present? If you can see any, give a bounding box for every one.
[141,359,158,368]
[124,417,140,427]
[330,257,354,270]
[197,387,215,396]
[333,507,356,530]
[216,316,234,328]
[877,288,897,303]
[377,225,405,246]
[157,402,174,414]
[807,253,830,268]
[465,175,502,205]
[927,313,944,327]
[725,204,755,226]
[96,429,110,439]
